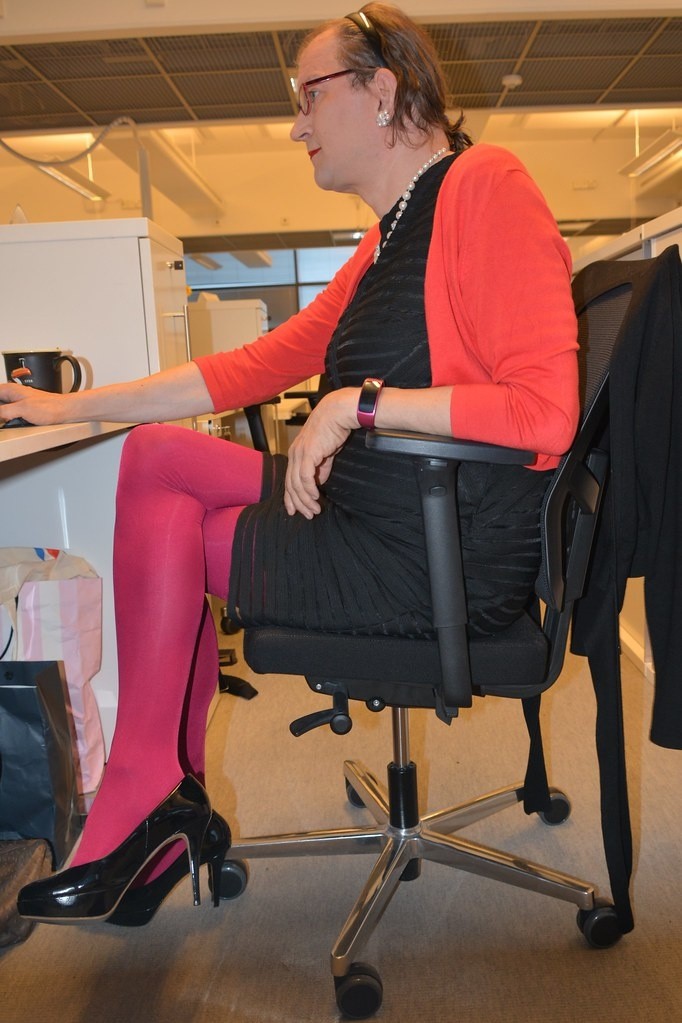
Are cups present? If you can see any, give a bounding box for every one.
[2,348,82,395]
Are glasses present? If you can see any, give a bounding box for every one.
[297,66,376,115]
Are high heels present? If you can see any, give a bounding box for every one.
[106,809,231,927]
[16,772,213,924]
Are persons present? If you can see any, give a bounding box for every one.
[0,8,581,926]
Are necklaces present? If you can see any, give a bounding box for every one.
[373,147,448,265]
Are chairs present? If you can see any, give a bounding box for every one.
[241,245,681,1020]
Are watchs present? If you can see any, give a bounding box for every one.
[357,377,384,429]
